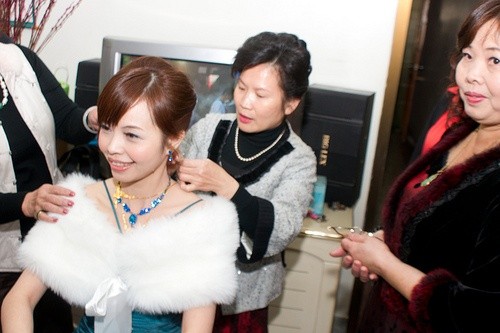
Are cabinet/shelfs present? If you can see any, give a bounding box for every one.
[268,203,353,333]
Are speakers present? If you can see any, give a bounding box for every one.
[75,59,101,111]
[303,84,375,208]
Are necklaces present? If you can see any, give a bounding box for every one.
[234,121,287,161]
[419,122,499,186]
[114,175,174,230]
[0,75,8,109]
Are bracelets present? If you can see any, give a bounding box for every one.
[82,106,101,134]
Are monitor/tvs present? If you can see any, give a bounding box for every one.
[98,36,305,140]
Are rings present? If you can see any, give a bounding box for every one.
[35,210,43,219]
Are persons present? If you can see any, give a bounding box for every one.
[0,31,101,333]
[169,31,318,333]
[1,55,242,333]
[328,1,500,333]
[183,72,238,127]
